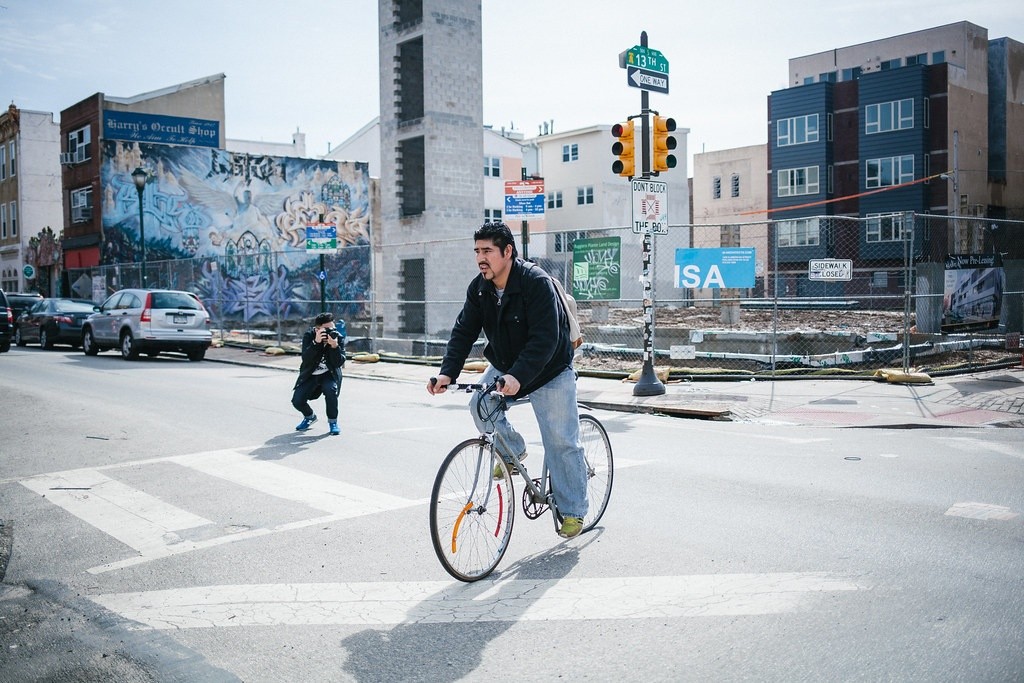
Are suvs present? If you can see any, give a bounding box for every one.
[82,288,213,361]
[5,292,43,342]
[0,289,13,352]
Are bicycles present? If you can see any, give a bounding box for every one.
[429,376,614,582]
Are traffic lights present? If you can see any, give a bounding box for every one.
[653,116,677,171]
[611,120,636,176]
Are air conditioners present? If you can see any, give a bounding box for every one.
[60,152,75,165]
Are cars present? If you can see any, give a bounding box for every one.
[13,297,100,350]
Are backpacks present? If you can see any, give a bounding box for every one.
[519,262,585,350]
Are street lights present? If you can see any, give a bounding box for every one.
[131,168,148,288]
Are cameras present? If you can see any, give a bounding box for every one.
[321,328,337,339]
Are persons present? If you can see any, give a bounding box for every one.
[427,221,590,537]
[292,313,346,436]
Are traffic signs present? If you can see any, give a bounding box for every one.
[627,64,669,95]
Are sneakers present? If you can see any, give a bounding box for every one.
[494,448,528,481]
[296,413,318,431]
[559,511,583,538]
[330,421,339,435]
